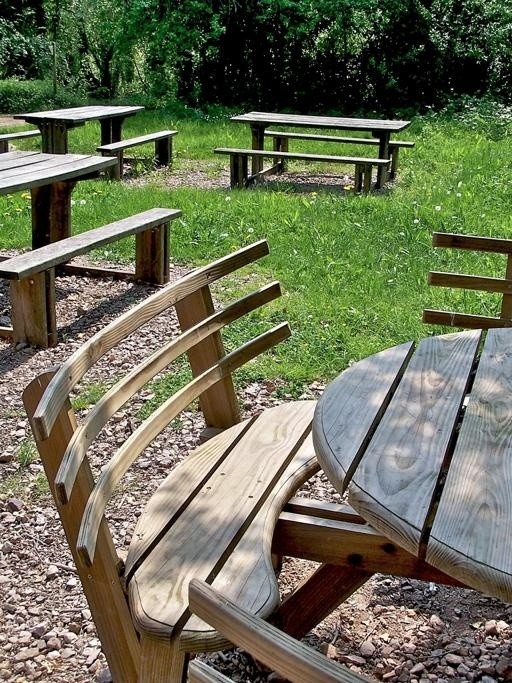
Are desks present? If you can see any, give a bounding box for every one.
[314,327,511,604]
[13,105,145,153]
[0,149,118,249]
[231,111,412,188]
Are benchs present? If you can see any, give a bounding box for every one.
[0,128,42,153]
[265,130,415,180]
[20,239,323,683]
[215,146,390,192]
[97,131,179,179]
[422,230,512,326]
[0,208,184,347]
[187,577,371,683]
